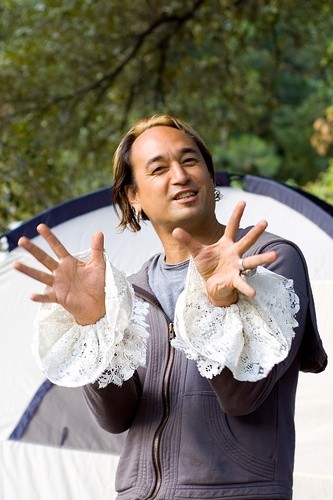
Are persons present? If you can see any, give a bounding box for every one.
[12,113,328,500]
